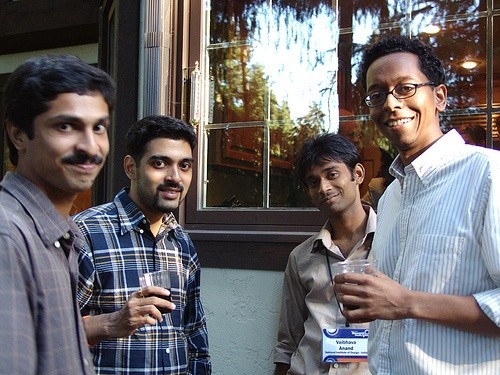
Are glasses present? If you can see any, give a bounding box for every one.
[363,82,435,108]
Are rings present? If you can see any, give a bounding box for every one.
[138,289,143,296]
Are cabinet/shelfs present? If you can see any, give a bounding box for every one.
[210,0,367,179]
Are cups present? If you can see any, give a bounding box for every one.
[332,259,378,324]
[139,269,172,315]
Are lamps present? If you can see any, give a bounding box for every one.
[210,67,215,124]
[184,61,202,133]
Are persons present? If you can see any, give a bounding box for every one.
[70,116,212,375]
[0,55,116,375]
[332,35,500,375]
[274,133,377,375]
